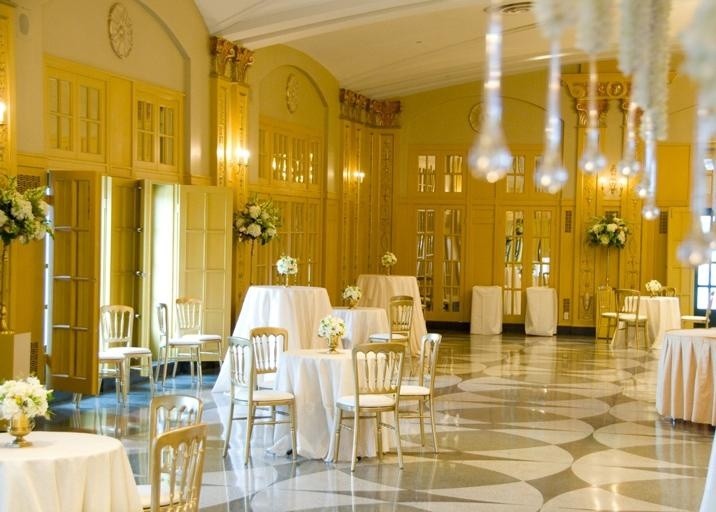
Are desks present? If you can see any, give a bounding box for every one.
[470,286,503,335]
[525,286,557,336]
[0,431,143,511]
[655,327,716,426]
[1,330,31,381]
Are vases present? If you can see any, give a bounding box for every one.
[0,304,14,335]
[7,415,33,448]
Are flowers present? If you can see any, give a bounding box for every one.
[645,280,662,291]
[0,167,61,251]
[380,250,398,270]
[232,190,282,246]
[587,213,631,251]
[0,376,54,420]
[275,254,300,278]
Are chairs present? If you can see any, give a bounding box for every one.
[595,286,714,353]
[135,395,207,510]
[73,297,220,409]
[212,275,442,471]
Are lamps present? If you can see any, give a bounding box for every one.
[355,171,365,185]
[467,1,715,267]
[234,146,250,178]
